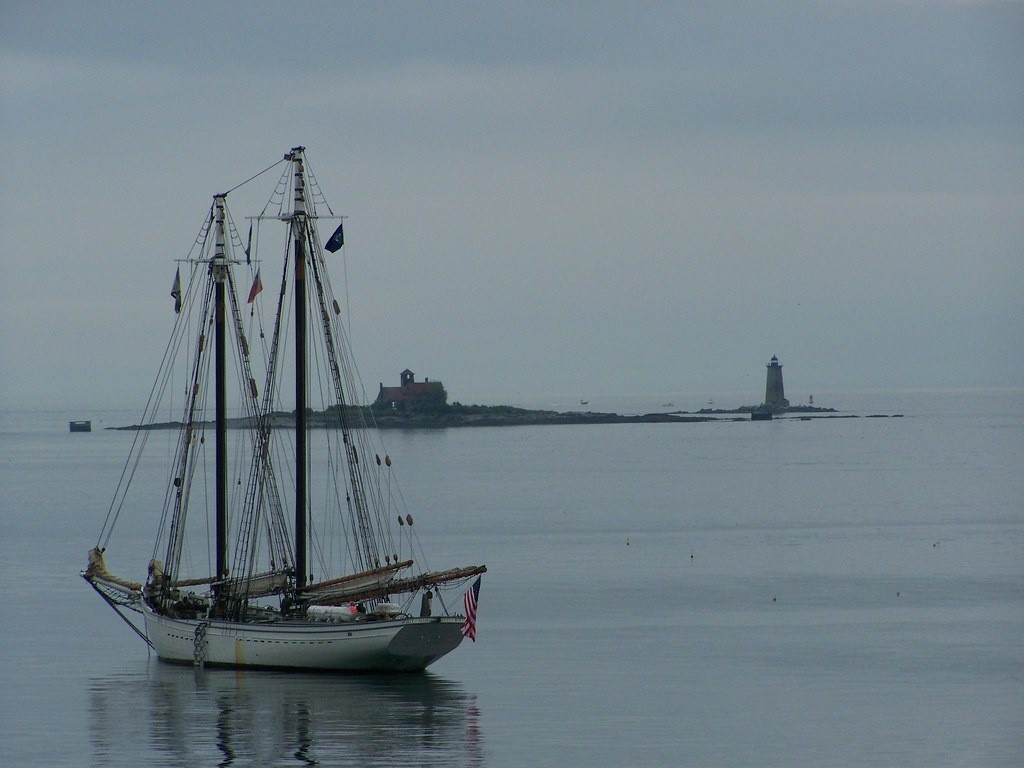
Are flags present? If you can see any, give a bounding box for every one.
[461,576,481,641]
[171,265,182,314]
[246,225,252,263]
[247,268,263,305]
[325,224,344,254]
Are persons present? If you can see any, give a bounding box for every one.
[281,596,289,613]
[347,602,366,613]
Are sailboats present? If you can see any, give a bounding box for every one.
[73,140,490,671]
